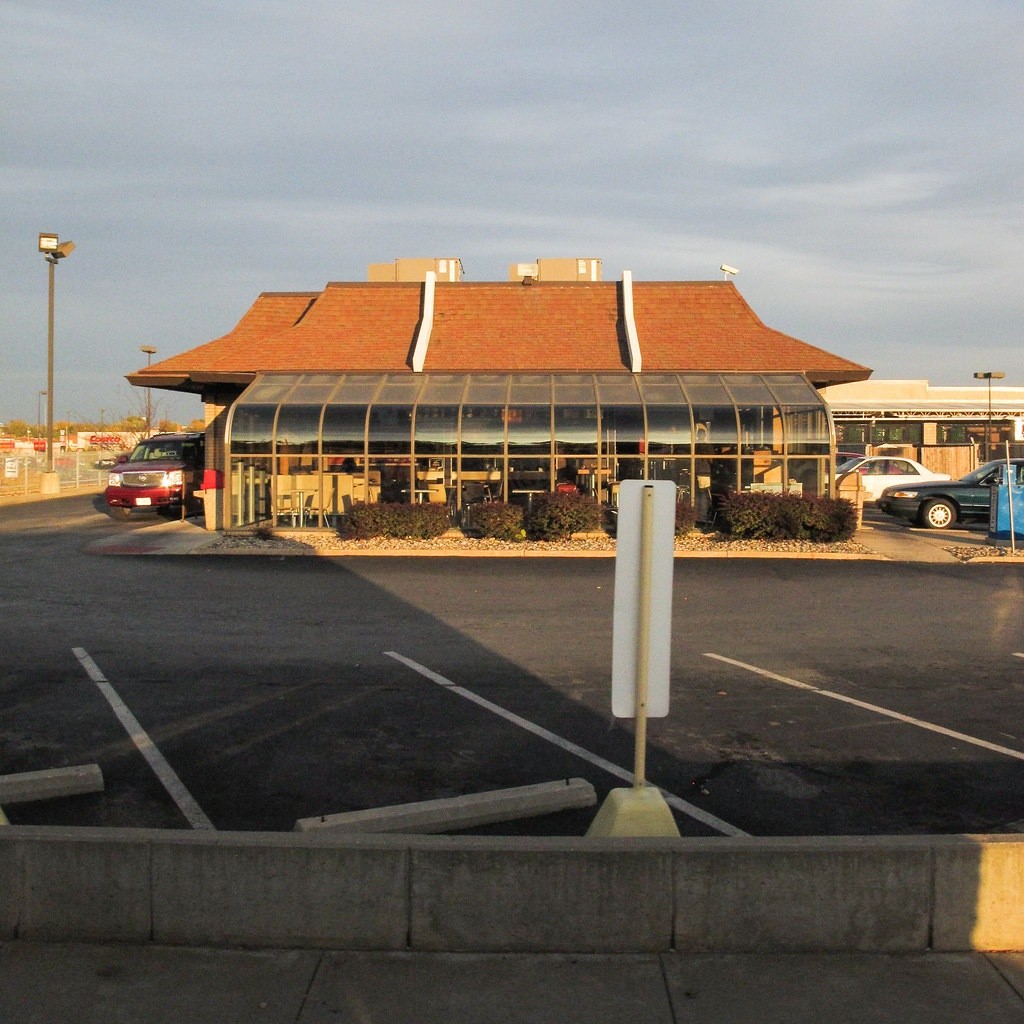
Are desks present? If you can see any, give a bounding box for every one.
[511,489,547,509]
[284,489,319,528]
[401,489,439,505]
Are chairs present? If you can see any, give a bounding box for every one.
[304,486,336,528]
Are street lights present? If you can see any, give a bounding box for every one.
[38,389,48,435]
[139,344,157,440]
[37,230,76,486]
[974,371,1006,466]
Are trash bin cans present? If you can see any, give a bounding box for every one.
[192,468,225,531]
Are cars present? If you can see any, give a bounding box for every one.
[824,455,951,505]
[876,457,1024,530]
[834,451,903,474]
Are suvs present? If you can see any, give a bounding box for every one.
[105,431,205,516]
